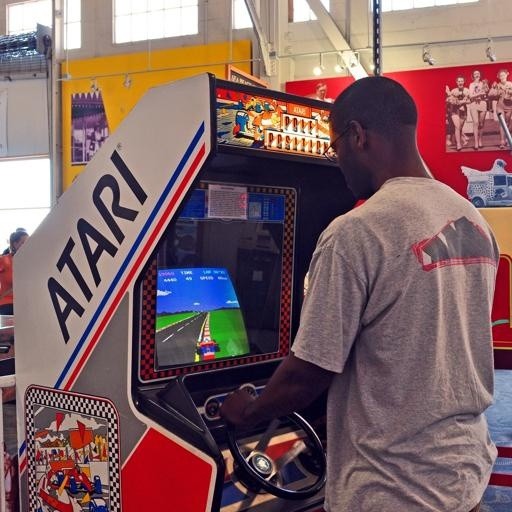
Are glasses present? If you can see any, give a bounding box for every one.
[323,127,351,163]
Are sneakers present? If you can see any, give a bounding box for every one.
[456,141,508,151]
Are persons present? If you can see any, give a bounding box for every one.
[446,76,471,150]
[0,226,30,314]
[488,70,511,151]
[216,75,500,512]
[468,70,491,150]
[84,122,108,162]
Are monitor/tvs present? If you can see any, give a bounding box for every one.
[153,264,253,369]
